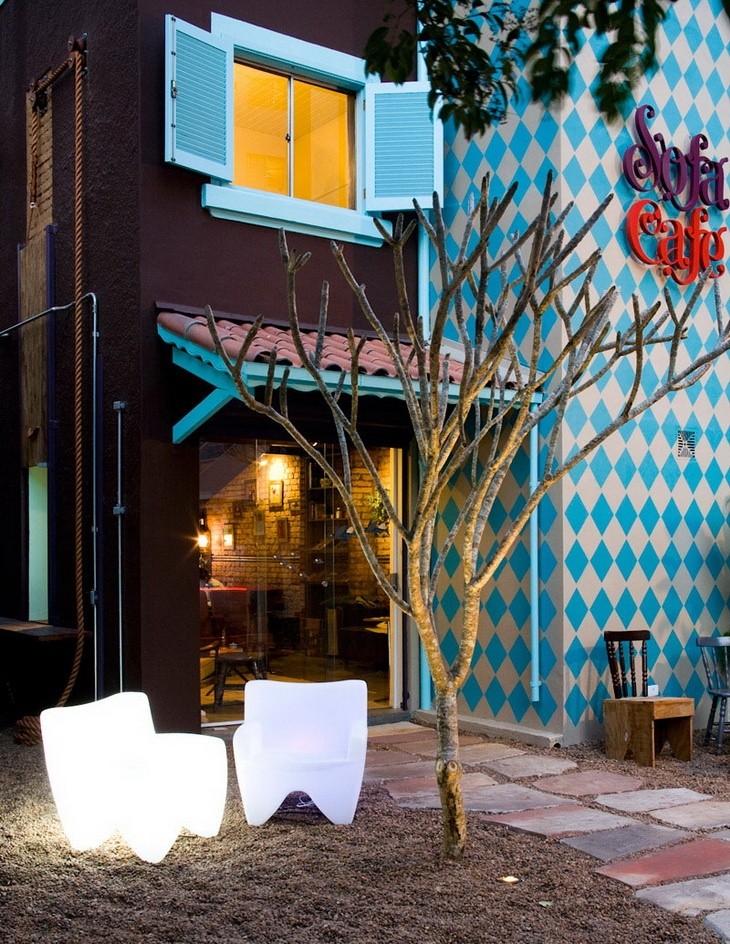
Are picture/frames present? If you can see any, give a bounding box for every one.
[222,478,300,552]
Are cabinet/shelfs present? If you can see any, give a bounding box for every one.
[304,452,351,618]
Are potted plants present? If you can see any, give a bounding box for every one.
[364,486,390,538]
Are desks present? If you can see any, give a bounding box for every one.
[210,647,269,712]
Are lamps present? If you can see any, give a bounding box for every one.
[197,515,211,553]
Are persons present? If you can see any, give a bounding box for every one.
[199,554,226,617]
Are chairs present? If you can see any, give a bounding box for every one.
[232,679,369,828]
[695,635,730,757]
[37,691,229,864]
[598,628,695,768]
[199,583,286,658]
